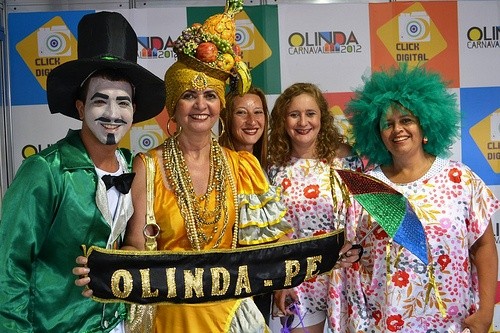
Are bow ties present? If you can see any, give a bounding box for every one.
[101,172,136,194]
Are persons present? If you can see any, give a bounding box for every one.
[260,82,368,332]
[214,81,274,329]
[0,60,141,333]
[71,57,362,333]
[323,58,500,333]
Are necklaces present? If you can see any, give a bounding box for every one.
[160,128,239,251]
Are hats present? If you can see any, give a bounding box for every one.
[164,0,252,122]
[46,10,166,124]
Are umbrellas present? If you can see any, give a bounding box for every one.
[332,164,432,270]
